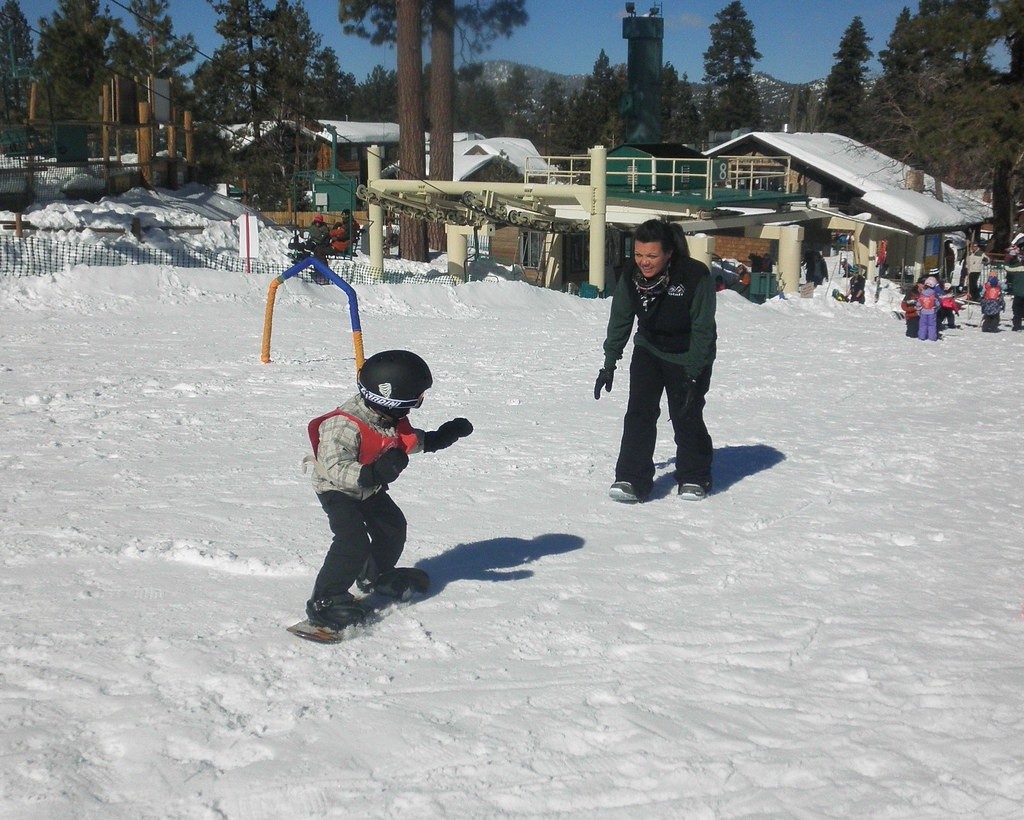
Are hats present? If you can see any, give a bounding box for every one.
[943,283,951,291]
[917,277,927,284]
[314,215,323,223]
[990,272,999,278]
[850,268,858,273]
[990,277,999,287]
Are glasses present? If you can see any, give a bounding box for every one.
[414,392,424,409]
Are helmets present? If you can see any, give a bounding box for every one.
[841,258,847,263]
[925,276,938,288]
[359,350,432,417]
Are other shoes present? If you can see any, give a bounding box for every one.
[356,567,428,598]
[306,596,374,630]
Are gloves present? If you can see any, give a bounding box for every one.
[359,448,409,488]
[594,367,614,399]
[679,379,701,414]
[424,418,473,453]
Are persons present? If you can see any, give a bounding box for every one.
[810,252,828,288]
[731,264,750,300]
[979,272,1005,334]
[762,253,772,273]
[748,253,762,273]
[715,275,727,292]
[1006,247,1024,331]
[965,241,991,302]
[594,219,717,502]
[295,209,360,261]
[849,267,865,304]
[959,238,973,292]
[301,350,473,632]
[901,268,959,342]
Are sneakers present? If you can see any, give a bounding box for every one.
[680,482,705,501]
[607,481,645,501]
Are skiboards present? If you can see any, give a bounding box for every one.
[278,248,314,271]
